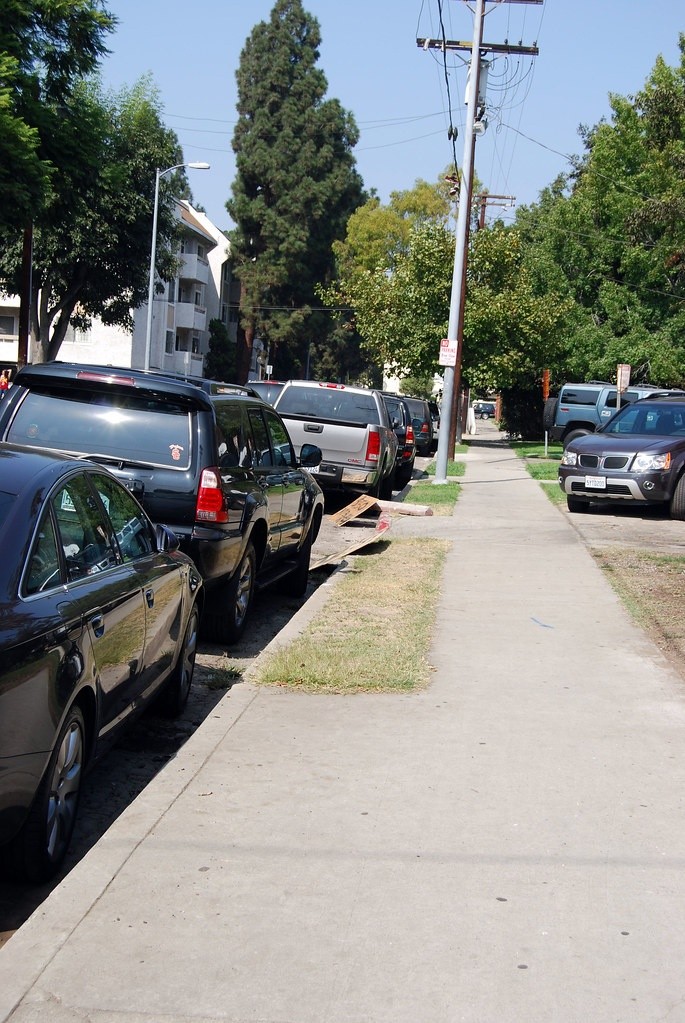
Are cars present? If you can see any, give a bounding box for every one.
[472,403,495,420]
[1,440,208,886]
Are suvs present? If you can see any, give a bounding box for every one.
[557,389,685,519]
[240,379,441,501]
[0,359,326,646]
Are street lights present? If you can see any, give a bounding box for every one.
[144,162,211,371]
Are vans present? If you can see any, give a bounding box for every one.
[553,380,685,451]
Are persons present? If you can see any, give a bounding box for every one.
[0,370,9,399]
